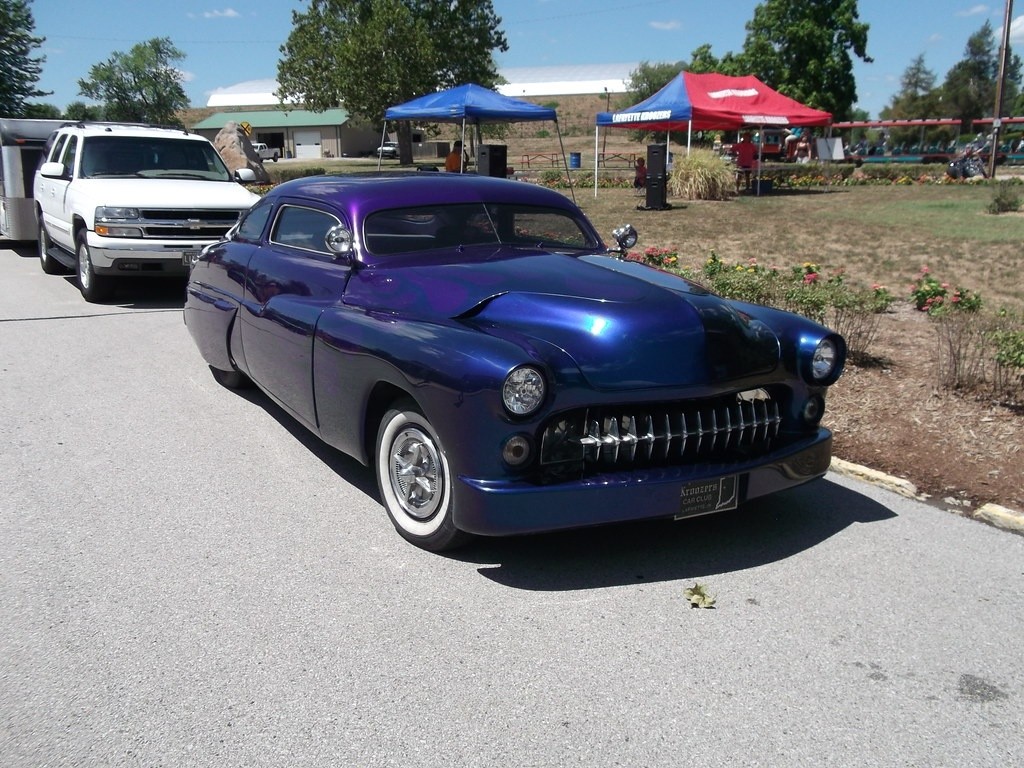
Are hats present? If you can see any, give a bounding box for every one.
[453,141,466,147]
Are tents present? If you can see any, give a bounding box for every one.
[594,69,834,197]
[376,82,577,207]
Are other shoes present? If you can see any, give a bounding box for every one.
[744,189,751,192]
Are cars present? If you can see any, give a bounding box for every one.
[376,142,401,159]
[179,170,853,556]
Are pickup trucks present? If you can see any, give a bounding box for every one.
[252,143,280,162]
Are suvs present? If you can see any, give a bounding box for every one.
[31,120,268,304]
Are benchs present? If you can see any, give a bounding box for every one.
[520,152,558,169]
[598,152,640,168]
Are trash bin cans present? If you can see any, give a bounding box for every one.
[570,152,581,168]
[286,151,292,158]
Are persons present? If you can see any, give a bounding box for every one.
[732,126,890,195]
[445,140,469,173]
[970,126,1024,156]
[634,157,651,210]
